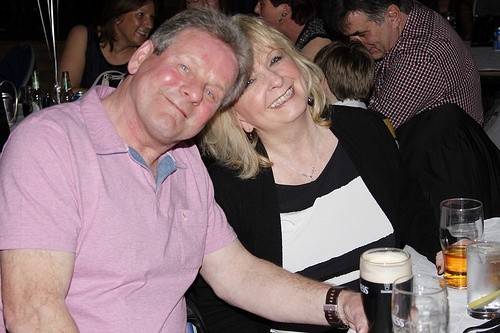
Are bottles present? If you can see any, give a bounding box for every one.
[32,70,45,109]
[21,86,33,117]
[61,71,74,102]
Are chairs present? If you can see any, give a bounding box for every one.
[1,44,36,93]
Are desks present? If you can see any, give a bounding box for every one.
[270,217,500,333]
[469,46,500,77]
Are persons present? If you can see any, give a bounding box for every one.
[0,1,484,333]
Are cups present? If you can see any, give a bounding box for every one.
[360,248,413,333]
[440,198,484,289]
[392,275,449,333]
[467,242,500,319]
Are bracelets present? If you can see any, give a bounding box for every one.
[325,284,348,330]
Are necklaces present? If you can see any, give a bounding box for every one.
[261,126,332,181]
